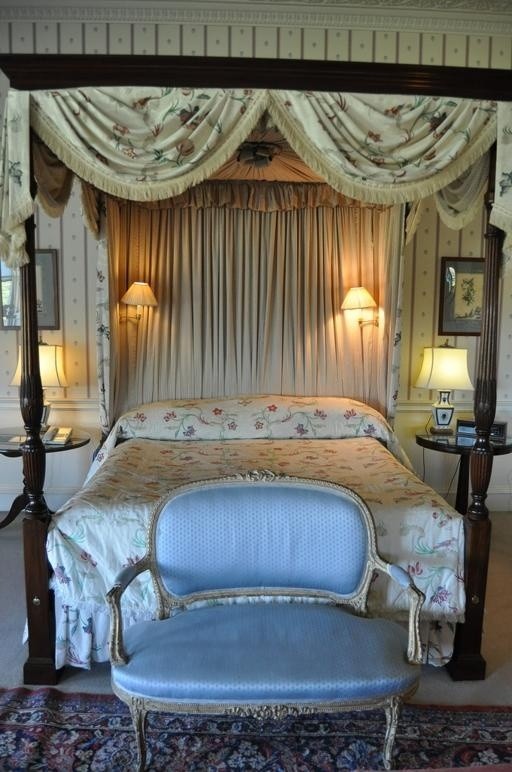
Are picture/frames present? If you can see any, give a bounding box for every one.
[437,255,486,336]
[1,247,59,331]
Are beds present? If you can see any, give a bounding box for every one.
[0,52,511,687]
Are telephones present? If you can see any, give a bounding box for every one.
[41,425,74,445]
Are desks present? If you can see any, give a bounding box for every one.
[0,422,92,534]
[414,425,512,516]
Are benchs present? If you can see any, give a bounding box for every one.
[105,467,427,769]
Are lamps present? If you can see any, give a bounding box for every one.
[118,280,157,321]
[9,341,70,432]
[414,338,473,433]
[339,287,381,329]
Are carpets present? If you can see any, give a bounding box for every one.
[0,682,510,771]
